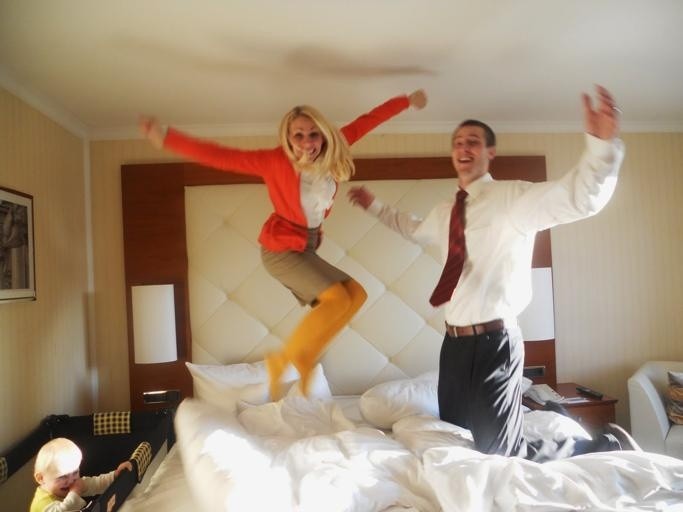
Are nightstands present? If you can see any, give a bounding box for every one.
[528,383,616,435]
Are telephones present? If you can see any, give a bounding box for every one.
[524,383,563,405]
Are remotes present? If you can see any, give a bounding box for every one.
[576,387,602,399]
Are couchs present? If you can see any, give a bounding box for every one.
[628,358,683,463]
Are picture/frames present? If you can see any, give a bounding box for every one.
[1,183,35,302]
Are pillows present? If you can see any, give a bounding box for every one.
[360,363,533,430]
[667,371,680,383]
[663,387,680,427]
[183,361,337,421]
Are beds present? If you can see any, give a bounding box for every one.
[0,409,177,512]
[107,156,682,511]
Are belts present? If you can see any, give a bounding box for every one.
[445,317,520,336]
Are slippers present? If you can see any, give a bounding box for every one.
[546,401,578,418]
[606,421,640,451]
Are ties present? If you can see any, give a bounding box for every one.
[428,190,468,307]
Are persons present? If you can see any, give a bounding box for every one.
[134,89,427,403]
[345,83,647,465]
[29,437,134,512]
